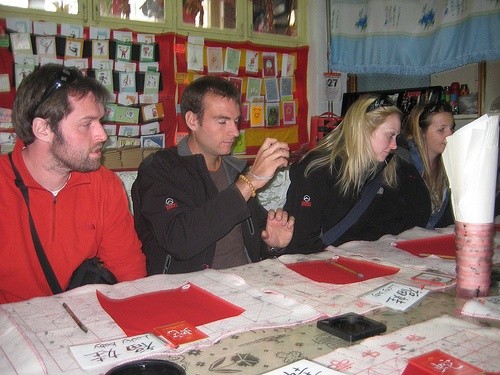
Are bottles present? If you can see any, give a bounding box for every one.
[460,83,470,96]
[442,86,451,104]
[406,96,414,111]
[449,82,460,114]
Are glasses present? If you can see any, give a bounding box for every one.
[365,94,395,113]
[420,98,448,119]
[34,66,83,114]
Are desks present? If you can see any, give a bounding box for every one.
[0,216,500,375]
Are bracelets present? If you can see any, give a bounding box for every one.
[238,174,256,197]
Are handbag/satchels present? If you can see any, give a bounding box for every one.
[66,255,118,291]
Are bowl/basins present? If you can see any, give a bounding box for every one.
[106,358,187,375]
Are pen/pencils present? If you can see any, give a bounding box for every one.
[419,253,456,259]
[63,303,88,333]
[330,261,364,278]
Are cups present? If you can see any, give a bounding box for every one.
[453,220,496,298]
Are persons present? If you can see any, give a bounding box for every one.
[1,62,147,305]
[283,93,420,254]
[131,74,294,277]
[397,100,456,228]
